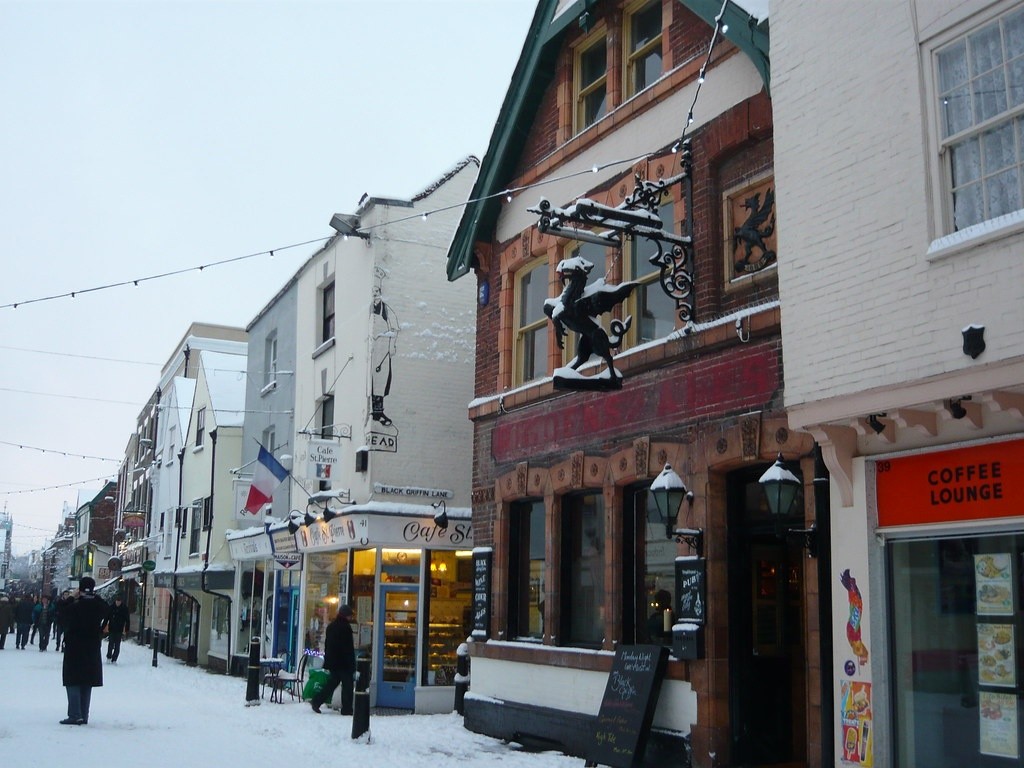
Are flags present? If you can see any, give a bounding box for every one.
[245,445,289,515]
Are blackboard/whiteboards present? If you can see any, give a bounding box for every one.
[584,645,672,768]
[674,556,707,624]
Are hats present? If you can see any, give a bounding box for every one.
[339,605,355,616]
[79,577,96,593]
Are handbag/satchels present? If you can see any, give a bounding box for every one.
[301,668,334,704]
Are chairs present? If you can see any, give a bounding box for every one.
[262,651,295,702]
[270,654,308,703]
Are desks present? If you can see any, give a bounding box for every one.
[261,658,285,703]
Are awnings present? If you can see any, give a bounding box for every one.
[92,575,122,592]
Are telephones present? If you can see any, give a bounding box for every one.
[470,552,491,641]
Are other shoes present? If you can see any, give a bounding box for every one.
[56,646,60,650]
[341,706,353,715]
[309,698,322,714]
[59,716,88,724]
[0,647,4,649]
[16,646,20,649]
[21,647,25,649]
[39,647,47,652]
[112,660,118,666]
[30,641,34,644]
[106,656,111,663]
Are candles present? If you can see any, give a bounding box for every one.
[664,609,672,632]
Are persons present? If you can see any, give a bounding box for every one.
[55,576,110,723]
[309,604,357,716]
[0,590,130,666]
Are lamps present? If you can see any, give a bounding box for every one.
[432,500,449,528]
[758,451,819,559]
[288,509,306,535]
[439,553,448,571]
[651,461,707,574]
[105,497,116,503]
[323,496,356,523]
[139,439,154,449]
[431,553,437,572]
[865,412,888,434]
[304,501,334,528]
[949,396,972,419]
[329,213,370,240]
